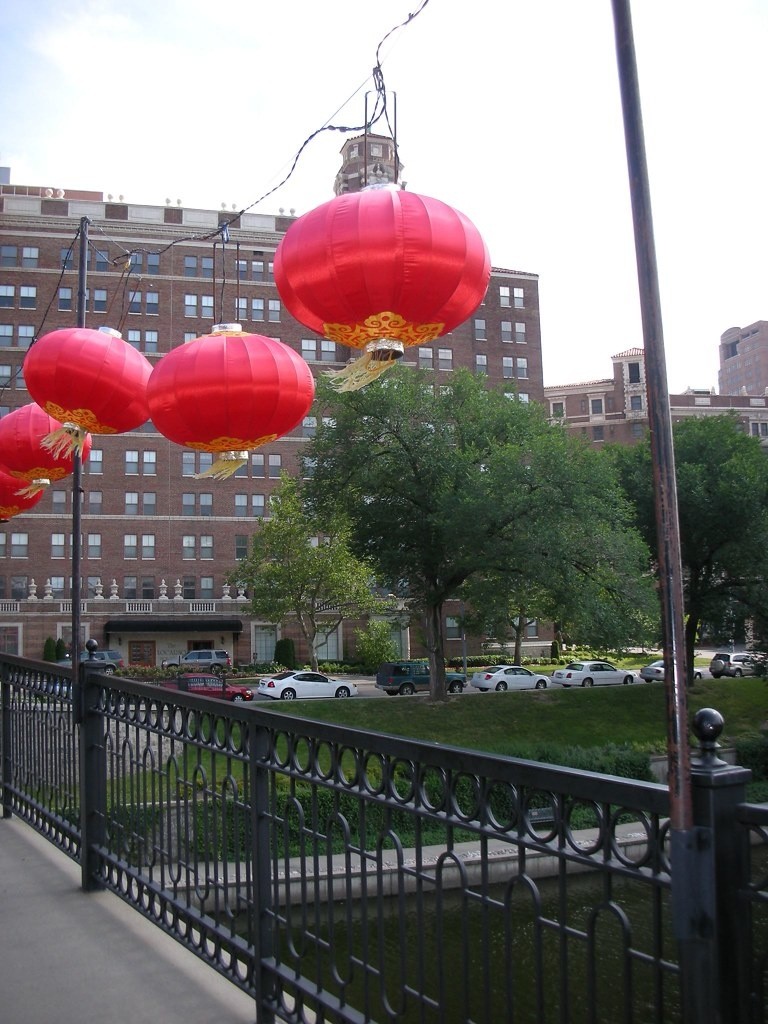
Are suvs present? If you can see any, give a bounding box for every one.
[709,653,763,679]
[59,649,125,677]
[162,649,232,675]
[375,660,468,696]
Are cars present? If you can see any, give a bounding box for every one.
[550,661,639,688]
[640,659,704,683]
[257,670,359,701]
[161,673,254,703]
[471,666,551,692]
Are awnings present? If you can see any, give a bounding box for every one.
[103,620,243,641]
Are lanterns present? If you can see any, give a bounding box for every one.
[272,181,491,393]
[22,326,155,461]
[146,323,315,481]
[0,401,92,524]
